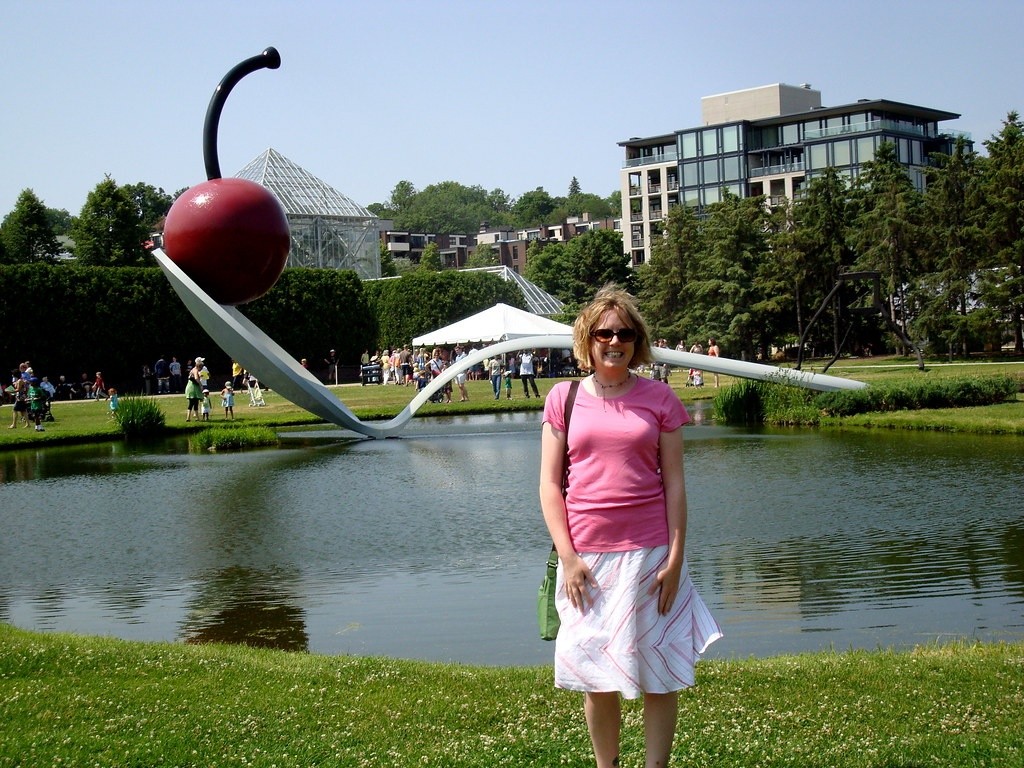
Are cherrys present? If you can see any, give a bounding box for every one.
[164,45,290,307]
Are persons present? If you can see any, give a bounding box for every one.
[92,372,109,401]
[142,354,193,395]
[425,349,442,370]
[675,340,686,372]
[539,284,724,768]
[441,361,453,403]
[454,346,469,402]
[231,359,249,391]
[185,356,235,421]
[515,348,540,399]
[302,359,307,368]
[570,352,581,377]
[8,360,50,432]
[40,373,96,401]
[360,344,539,387]
[324,349,339,381]
[108,388,119,418]
[503,371,512,400]
[690,368,703,389]
[651,338,671,383]
[489,354,506,400]
[708,338,719,387]
[689,342,703,354]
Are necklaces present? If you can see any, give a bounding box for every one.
[593,369,631,389]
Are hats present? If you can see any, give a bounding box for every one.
[11,368,22,379]
[27,377,38,383]
[330,349,335,353]
[195,357,205,362]
[225,381,231,389]
[201,389,210,393]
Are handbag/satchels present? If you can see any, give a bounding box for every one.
[537,567,561,640]
[189,369,200,386]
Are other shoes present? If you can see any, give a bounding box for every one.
[506,397,513,400]
[448,400,452,403]
[22,425,31,429]
[465,397,469,401]
[494,398,499,400]
[536,395,540,398]
[8,424,17,429]
[17,418,26,422]
[35,429,39,432]
[460,398,465,402]
[526,395,530,398]
[40,429,45,431]
[446,399,449,403]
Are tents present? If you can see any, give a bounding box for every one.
[412,303,576,355]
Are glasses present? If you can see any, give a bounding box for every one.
[589,328,639,343]
